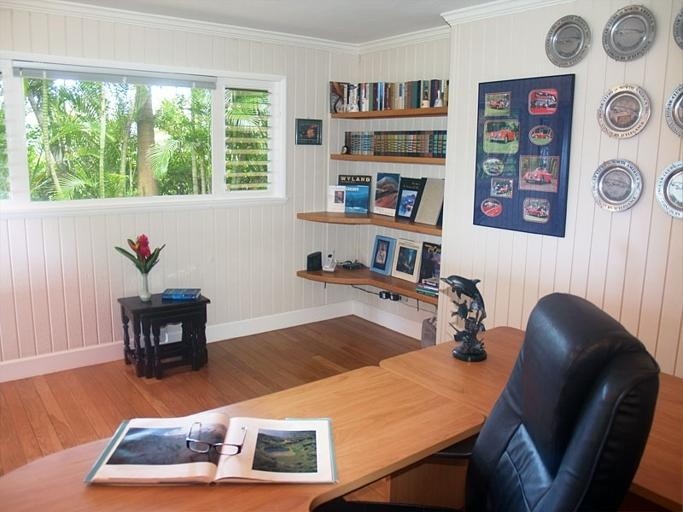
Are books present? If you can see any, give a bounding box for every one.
[86,413,335,487]
[328,78,448,298]
[162,287,202,300]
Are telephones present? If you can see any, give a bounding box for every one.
[323,248,337,273]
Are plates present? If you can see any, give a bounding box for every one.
[597,83,651,140]
[664,83,683,139]
[655,161,683,218]
[601,4,656,62]
[591,158,642,211]
[545,15,590,67]
[672,8,683,49]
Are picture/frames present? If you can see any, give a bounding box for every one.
[295,119,322,145]
[392,239,422,283]
[370,236,396,276]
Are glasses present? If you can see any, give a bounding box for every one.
[186,421,247,455]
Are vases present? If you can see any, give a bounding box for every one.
[139,273,151,302]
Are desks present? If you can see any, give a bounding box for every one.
[118,291,210,379]
[0,365,486,512]
[379,325,683,512]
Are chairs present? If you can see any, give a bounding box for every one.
[317,292,658,511]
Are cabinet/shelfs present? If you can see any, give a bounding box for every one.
[298,106,448,308]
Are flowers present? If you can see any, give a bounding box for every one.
[113,234,167,273]
